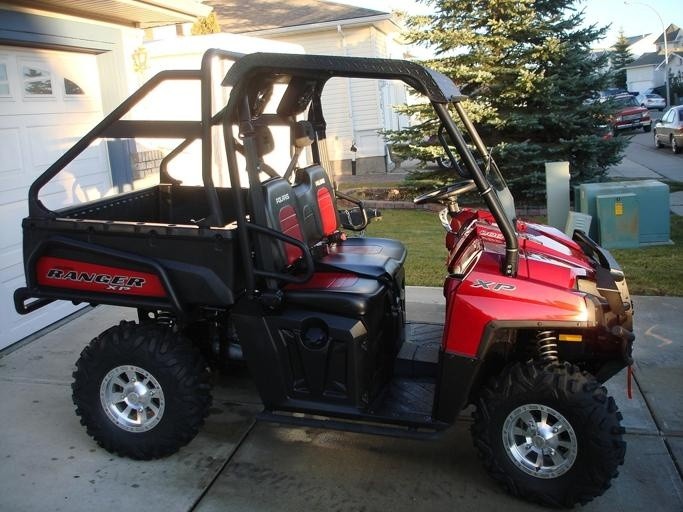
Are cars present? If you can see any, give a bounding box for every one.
[635,93,666,112]
[653,104,682,153]
[598,92,652,133]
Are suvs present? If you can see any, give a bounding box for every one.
[12,51,634,507]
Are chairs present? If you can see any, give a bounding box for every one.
[259,165,407,334]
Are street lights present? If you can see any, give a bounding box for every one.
[623,0,670,109]
